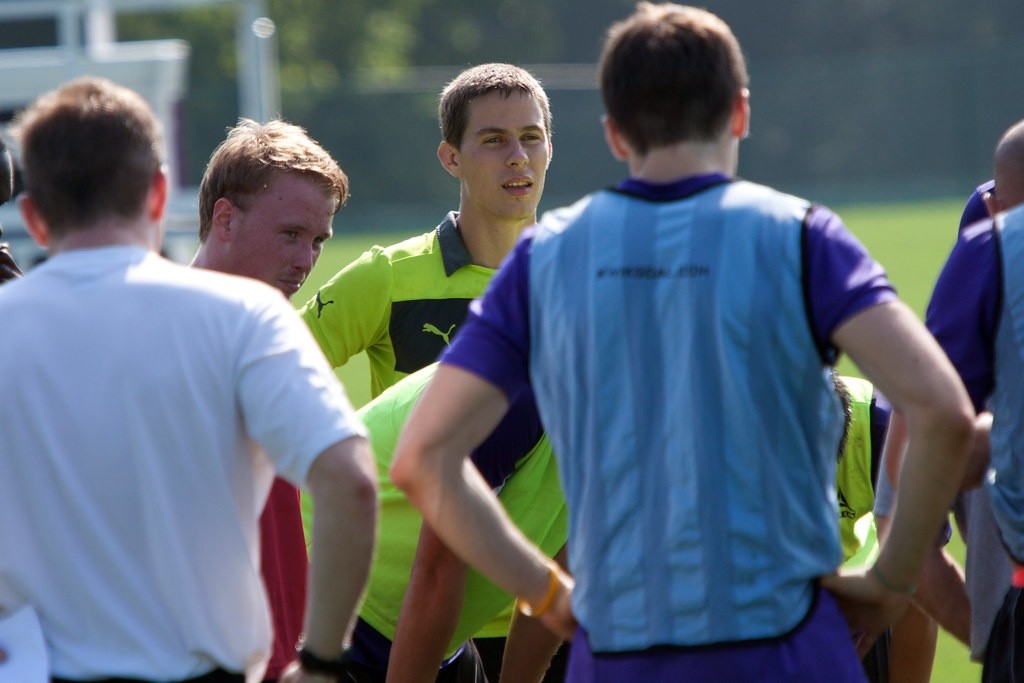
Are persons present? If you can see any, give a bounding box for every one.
[0,76,378,683]
[301,0,1024,683]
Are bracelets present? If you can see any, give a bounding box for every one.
[870,561,917,597]
[297,640,359,675]
[516,564,563,619]
